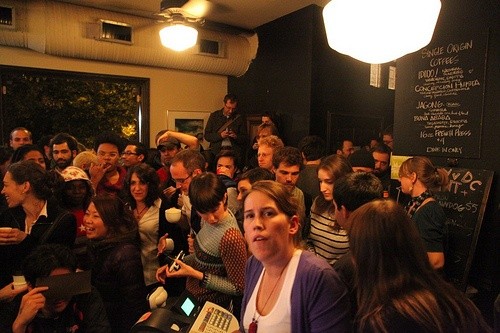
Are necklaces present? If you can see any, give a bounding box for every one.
[134,206,148,218]
[248,257,293,333]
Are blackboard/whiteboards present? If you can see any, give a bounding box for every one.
[391,24,493,159]
[425,166,496,292]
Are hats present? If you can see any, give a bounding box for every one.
[60,166,90,182]
[157,136,181,149]
[73,151,99,170]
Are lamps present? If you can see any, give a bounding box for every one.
[322,0,443,64]
[154,0,208,51]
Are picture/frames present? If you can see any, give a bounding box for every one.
[166,109,211,150]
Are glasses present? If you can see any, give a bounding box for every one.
[172,173,193,183]
[121,152,139,157]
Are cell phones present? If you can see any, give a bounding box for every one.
[169,250,184,273]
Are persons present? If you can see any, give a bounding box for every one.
[0,281,33,303]
[205,94,248,162]
[84,192,150,333]
[157,171,247,313]
[305,155,353,267]
[344,199,495,333]
[233,181,353,333]
[331,171,385,292]
[0,161,77,284]
[58,166,96,264]
[271,147,305,239]
[0,113,393,294]
[122,163,162,295]
[238,167,270,232]
[0,243,111,333]
[399,156,449,270]
